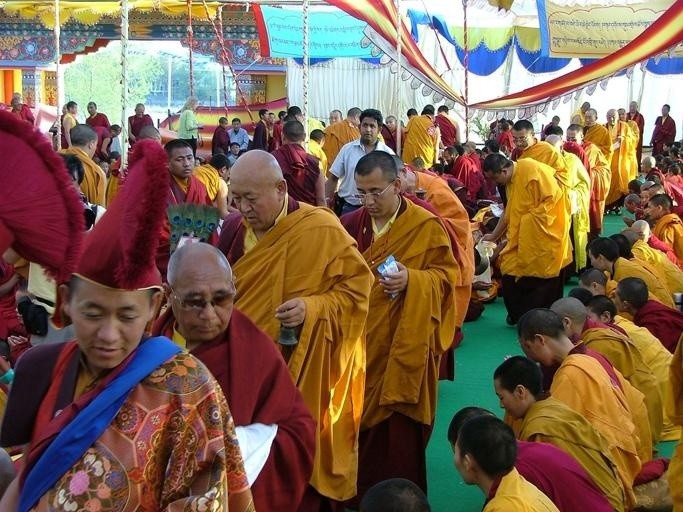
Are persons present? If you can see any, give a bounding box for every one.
[1,93,683,512]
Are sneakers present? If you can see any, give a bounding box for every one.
[604,208,612,215]
[506,314,517,327]
[614,206,622,216]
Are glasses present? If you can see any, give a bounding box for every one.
[352,180,395,201]
[169,281,237,313]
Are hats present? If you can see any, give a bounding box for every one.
[1,103,89,287]
[71,137,167,295]
